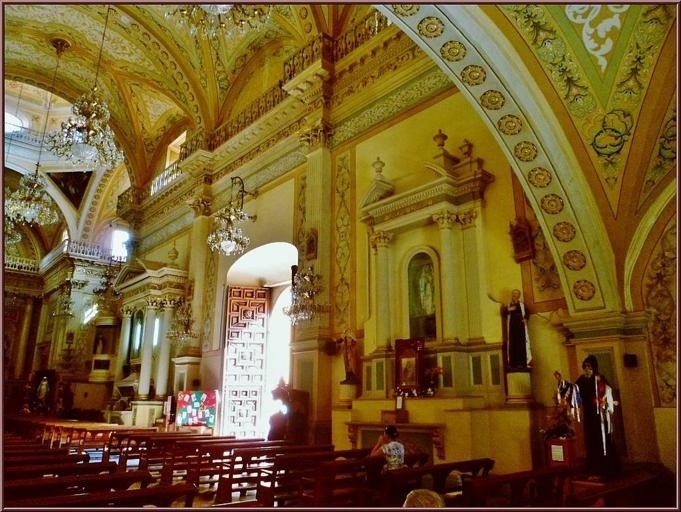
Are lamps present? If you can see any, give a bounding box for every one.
[42,3,133,172]
[206,175,255,260]
[281,261,331,327]
[164,297,202,352]
[3,37,71,233]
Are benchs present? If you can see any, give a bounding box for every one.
[1,397,679,510]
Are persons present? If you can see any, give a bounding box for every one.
[372,425,409,479]
[501,289,533,373]
[553,354,615,474]
[329,326,362,384]
